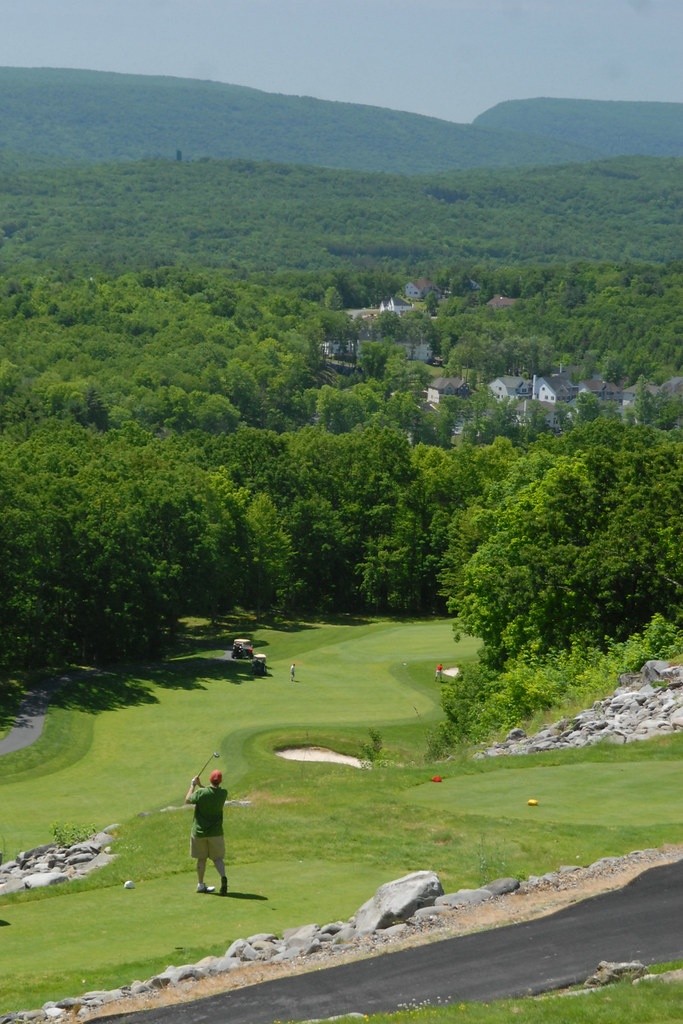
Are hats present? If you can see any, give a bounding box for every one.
[209,770,222,786]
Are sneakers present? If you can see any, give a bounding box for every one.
[219,876,227,894]
[197,883,215,892]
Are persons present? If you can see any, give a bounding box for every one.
[434,663,444,683]
[182,769,229,894]
[290,663,295,682]
